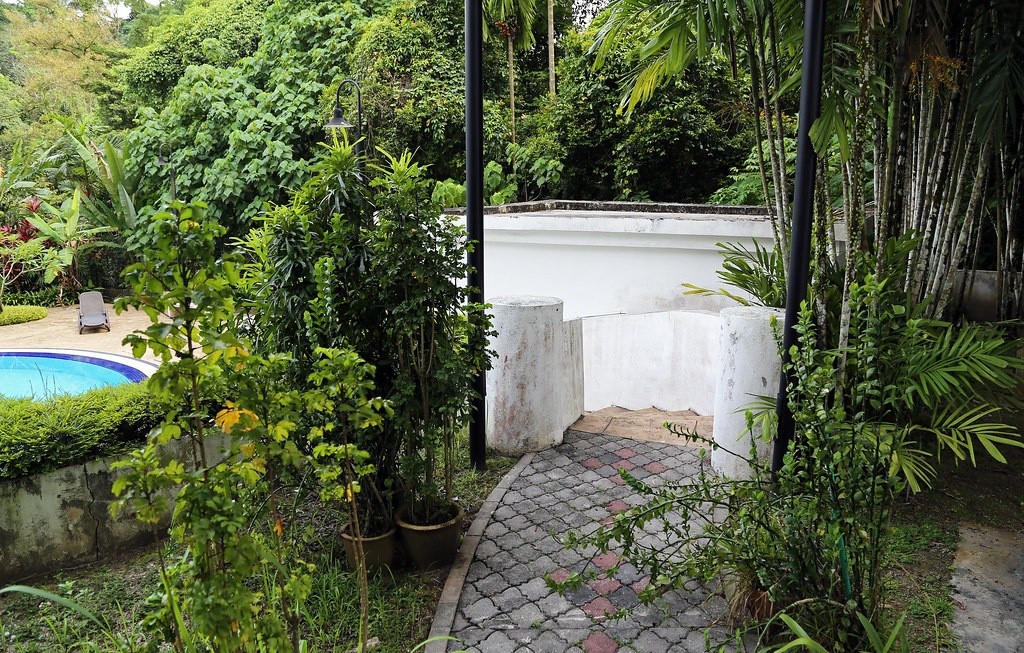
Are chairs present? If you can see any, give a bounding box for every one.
[78,292,110,334]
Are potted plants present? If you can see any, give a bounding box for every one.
[223,127,497,572]
[543,412,835,631]
